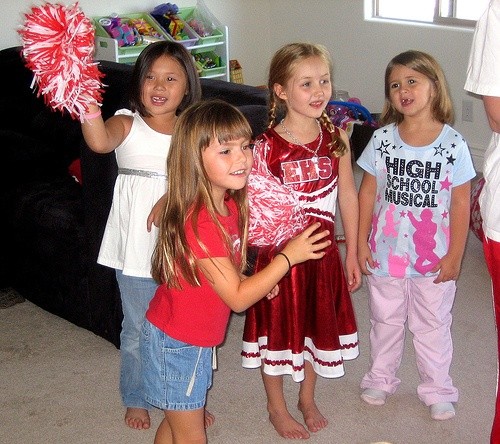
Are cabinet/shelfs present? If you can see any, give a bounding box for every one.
[94,0,230,82]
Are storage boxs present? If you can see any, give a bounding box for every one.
[91,7,225,84]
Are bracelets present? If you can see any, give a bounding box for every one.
[84,107,101,119]
[278,253,290,275]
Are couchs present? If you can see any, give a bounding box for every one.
[0,45,286,350]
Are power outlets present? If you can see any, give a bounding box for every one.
[462,101,473,122]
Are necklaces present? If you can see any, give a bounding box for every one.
[280,118,323,163]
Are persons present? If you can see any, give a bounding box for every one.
[354,51,477,421]
[139,100,332,444]
[463,0,500,444]
[80,42,216,429]
[247,43,362,439]
